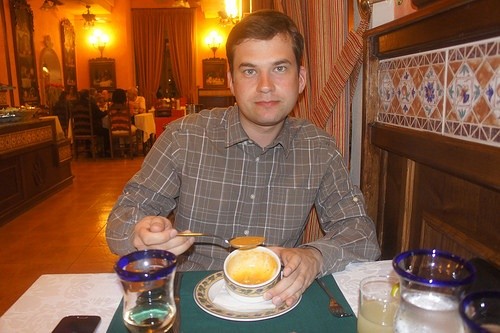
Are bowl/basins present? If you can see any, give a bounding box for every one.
[223,246,282,303]
[0,113,22,122]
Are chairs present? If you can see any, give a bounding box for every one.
[107,98,139,159]
[69,99,105,160]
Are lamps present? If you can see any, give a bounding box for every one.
[218,0,239,24]
[204,29,223,58]
[39,0,63,12]
[80,6,99,27]
[88,28,111,57]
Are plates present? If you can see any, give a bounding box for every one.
[193,270,302,322]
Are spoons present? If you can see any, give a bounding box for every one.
[176,232,266,250]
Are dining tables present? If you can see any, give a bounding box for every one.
[69,112,155,156]
[0,260,395,333]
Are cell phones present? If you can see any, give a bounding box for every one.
[51,315,101,333]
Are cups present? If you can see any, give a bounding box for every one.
[358,276,401,332]
[392,250,500,333]
[113,250,181,333]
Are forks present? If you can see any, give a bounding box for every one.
[315,278,352,318]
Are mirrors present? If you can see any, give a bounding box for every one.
[40,34,63,105]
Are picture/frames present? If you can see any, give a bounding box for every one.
[88,56,116,92]
[202,57,227,89]
[9,0,41,108]
[60,19,77,96]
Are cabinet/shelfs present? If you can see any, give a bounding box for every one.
[0,115,76,226]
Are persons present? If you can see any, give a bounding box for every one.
[54,86,146,157]
[106,8,380,308]
